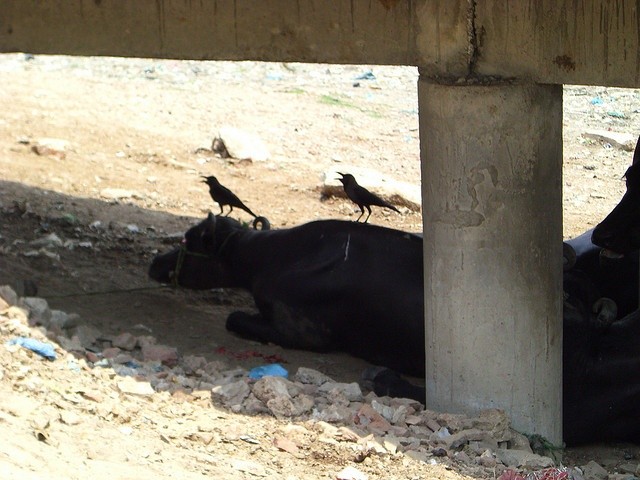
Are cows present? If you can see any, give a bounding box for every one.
[147,211,426,411]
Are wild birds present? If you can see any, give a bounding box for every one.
[198,175,259,219]
[335,171,404,224]
